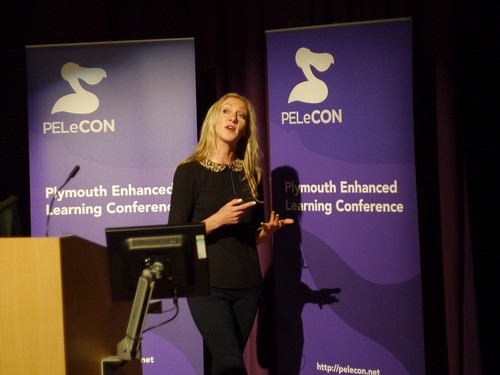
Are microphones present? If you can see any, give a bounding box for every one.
[46,165,80,236]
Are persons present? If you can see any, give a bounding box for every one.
[167,93,294,375]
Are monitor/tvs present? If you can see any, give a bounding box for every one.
[105,222,211,302]
